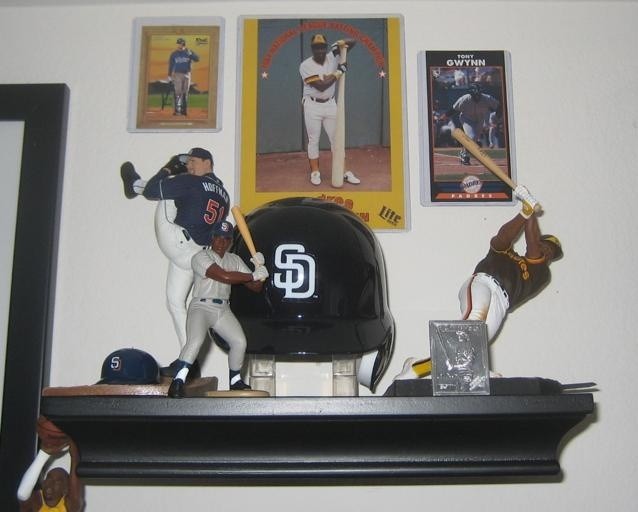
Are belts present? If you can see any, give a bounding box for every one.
[311,97,334,103]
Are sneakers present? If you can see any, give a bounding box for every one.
[343,172,360,184]
[461,157,469,165]
[311,172,322,186]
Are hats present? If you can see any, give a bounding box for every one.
[176,38,186,43]
[311,34,328,47]
[95,348,162,383]
[469,84,480,93]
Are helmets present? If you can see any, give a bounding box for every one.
[210,198,396,393]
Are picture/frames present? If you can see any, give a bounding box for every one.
[416,48,520,209]
[232,11,414,235]
[127,15,227,135]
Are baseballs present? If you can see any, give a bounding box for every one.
[462,175,481,194]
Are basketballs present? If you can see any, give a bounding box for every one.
[36,417,69,446]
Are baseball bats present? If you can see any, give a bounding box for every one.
[162,81,171,111]
[332,44,347,187]
[233,206,271,294]
[452,128,542,212]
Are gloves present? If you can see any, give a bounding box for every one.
[168,75,173,82]
[336,63,347,74]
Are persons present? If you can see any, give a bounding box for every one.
[167,221,269,398]
[392,184,565,385]
[167,38,199,115]
[432,68,503,166]
[120,147,230,379]
[298,34,361,185]
[15,435,84,512]
